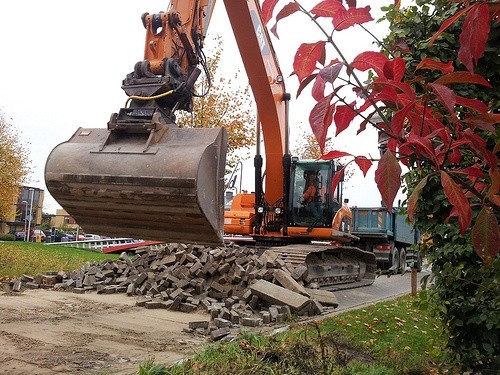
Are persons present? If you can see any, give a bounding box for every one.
[297,173,321,205]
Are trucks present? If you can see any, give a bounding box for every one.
[350,199,424,276]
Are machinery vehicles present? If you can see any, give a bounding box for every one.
[44,1,377,296]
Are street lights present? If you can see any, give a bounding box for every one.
[22,201,27,242]
[26,189,35,242]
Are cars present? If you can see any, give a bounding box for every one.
[14,228,112,246]
